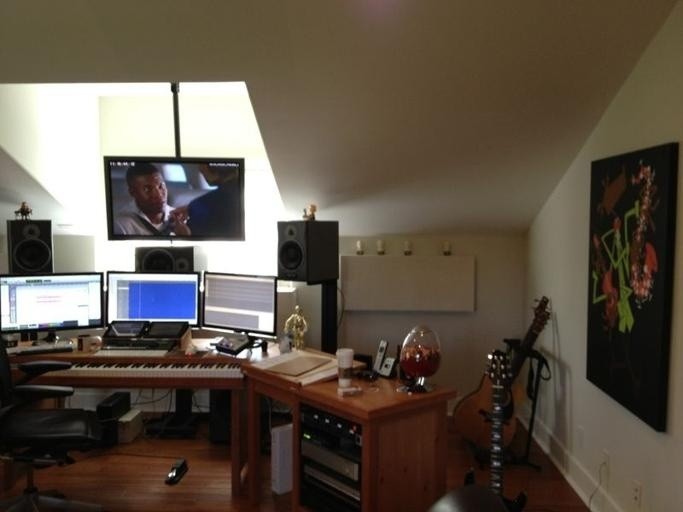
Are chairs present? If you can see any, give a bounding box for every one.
[1,337,101,509]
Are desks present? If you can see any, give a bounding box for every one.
[2,335,280,509]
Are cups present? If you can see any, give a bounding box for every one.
[77,334,102,353]
[336,348,354,388]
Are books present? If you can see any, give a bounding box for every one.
[252,348,368,387]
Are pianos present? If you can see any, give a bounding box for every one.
[9,359,248,379]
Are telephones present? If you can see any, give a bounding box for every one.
[372,339,389,373]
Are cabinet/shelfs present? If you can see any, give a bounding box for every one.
[240,346,455,511]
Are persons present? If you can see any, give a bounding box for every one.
[284,303,308,351]
[168,164,238,237]
[110,161,179,235]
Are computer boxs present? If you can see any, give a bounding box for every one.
[97,392,130,444]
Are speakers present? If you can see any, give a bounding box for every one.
[277,221,340,282]
[135,246,193,274]
[275,279,337,354]
[7,219,52,274]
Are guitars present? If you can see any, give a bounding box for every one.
[452,295,552,451]
[463,349,528,512]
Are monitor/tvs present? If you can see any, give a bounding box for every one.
[204,271,278,348]
[106,271,202,331]
[0,273,105,356]
[105,156,245,240]
